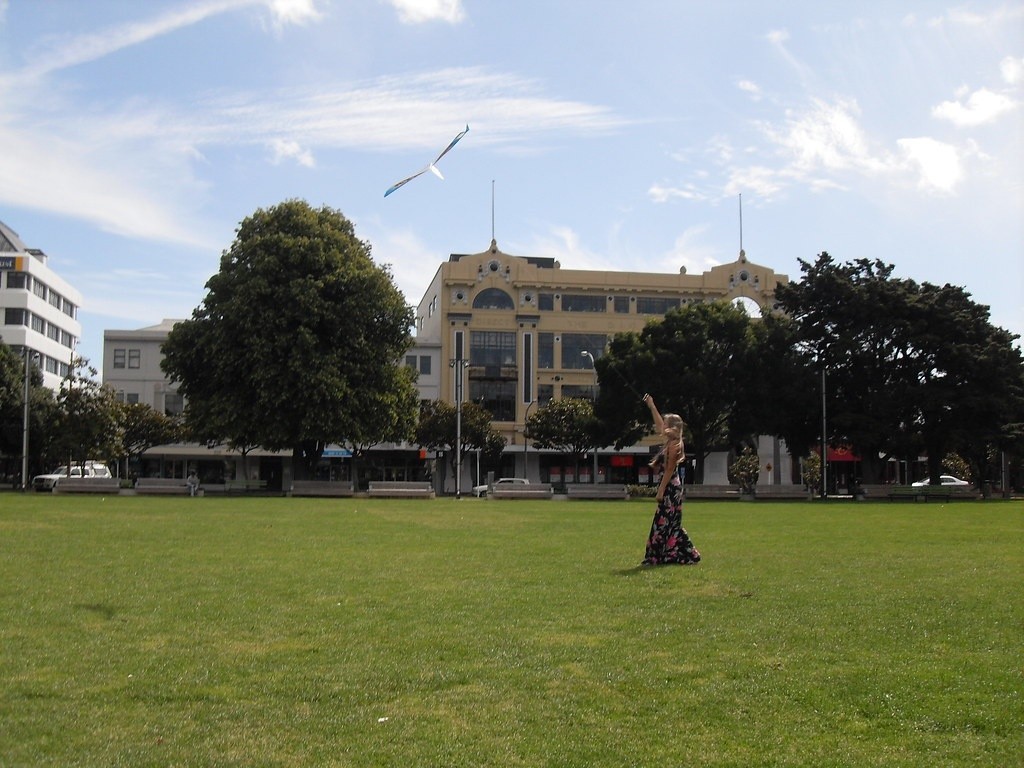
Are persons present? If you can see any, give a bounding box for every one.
[641,393,701,565]
[186,470,199,496]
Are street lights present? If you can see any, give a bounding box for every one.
[525,398,537,478]
[822,360,840,499]
[580,349,599,490]
[448,356,471,500]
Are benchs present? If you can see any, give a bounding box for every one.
[367,482,435,500]
[135,478,200,496]
[290,480,355,498]
[684,483,743,501]
[921,485,977,503]
[859,485,918,503]
[52,477,122,496]
[224,479,269,497]
[566,483,628,501]
[492,483,555,500]
[754,485,810,501]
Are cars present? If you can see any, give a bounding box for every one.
[912,473,970,488]
[472,477,529,498]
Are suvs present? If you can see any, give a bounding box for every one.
[31,460,112,493]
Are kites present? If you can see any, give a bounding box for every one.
[383,121,469,197]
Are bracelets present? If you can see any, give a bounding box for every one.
[652,405,655,409]
